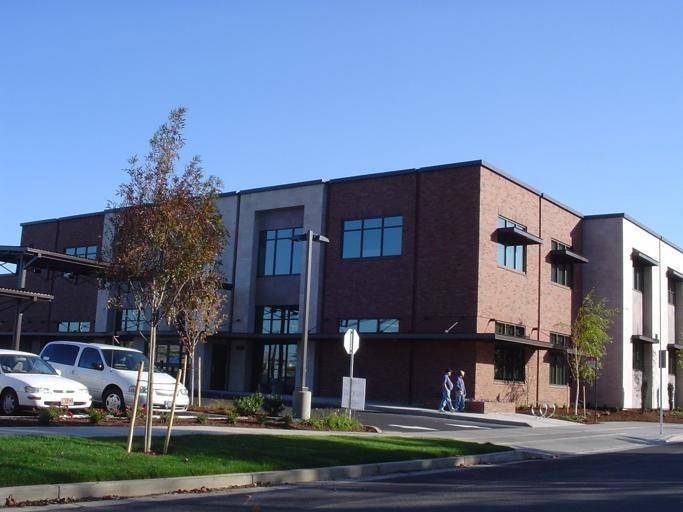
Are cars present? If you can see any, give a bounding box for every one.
[0,348,92,416]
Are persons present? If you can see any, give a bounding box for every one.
[451,369,466,412]
[437,367,458,412]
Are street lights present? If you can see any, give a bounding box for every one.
[292,229,330,421]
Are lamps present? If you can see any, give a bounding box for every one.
[96,270,107,291]
[57,272,75,281]
[33,264,42,274]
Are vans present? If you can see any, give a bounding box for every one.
[33,340,190,416]
